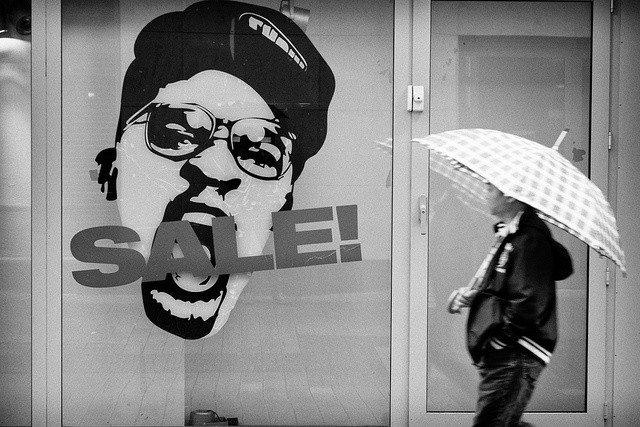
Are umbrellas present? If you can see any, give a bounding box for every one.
[410,126,626,314]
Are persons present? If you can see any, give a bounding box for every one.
[449,179,573,426]
